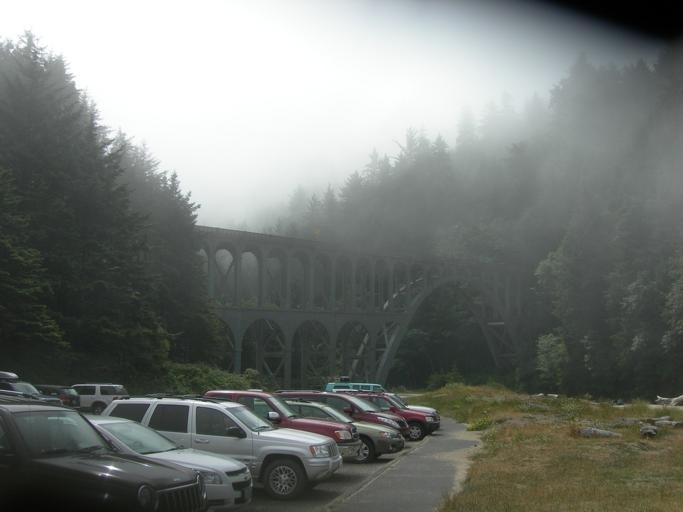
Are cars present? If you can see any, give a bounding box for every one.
[22,414,252,506]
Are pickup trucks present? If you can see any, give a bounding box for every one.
[203,389,363,458]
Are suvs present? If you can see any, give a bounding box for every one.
[33,384,81,411]
[71,383,130,415]
[100,393,342,500]
[253,382,441,463]
[1,389,208,512]
[1,371,64,406]
